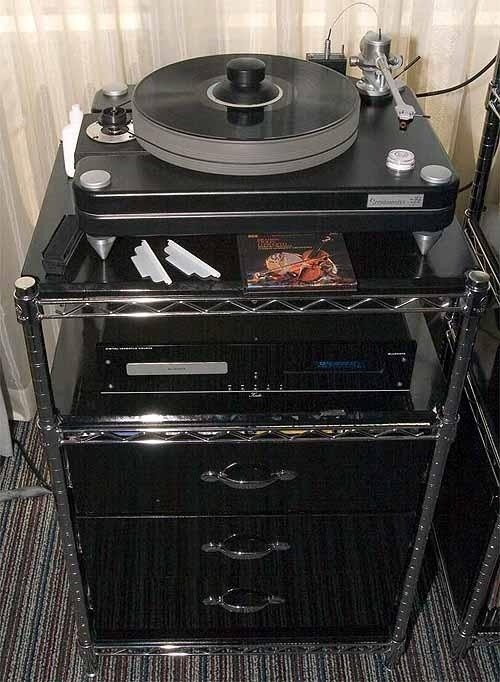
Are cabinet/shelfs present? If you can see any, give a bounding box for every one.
[63,439,437,671]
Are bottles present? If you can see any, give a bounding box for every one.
[62,104,83,178]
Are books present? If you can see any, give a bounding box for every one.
[237,231,359,291]
